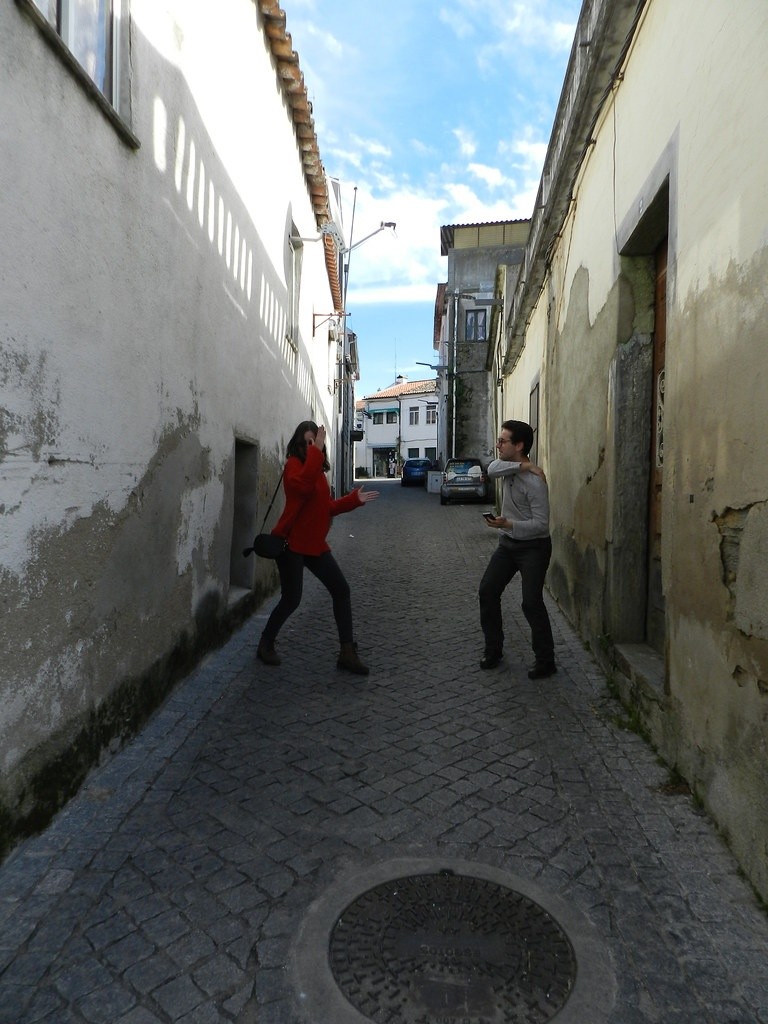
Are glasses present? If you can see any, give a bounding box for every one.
[498,438,512,446]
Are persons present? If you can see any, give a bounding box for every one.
[255,421,380,675]
[478,420,558,680]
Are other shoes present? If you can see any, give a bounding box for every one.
[480,648,504,669]
[528,652,558,679]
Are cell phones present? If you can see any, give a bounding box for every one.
[482,512,495,521]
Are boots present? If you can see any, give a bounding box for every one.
[256,636,281,666]
[331,643,370,676]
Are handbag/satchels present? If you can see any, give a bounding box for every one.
[243,534,288,559]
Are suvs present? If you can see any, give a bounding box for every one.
[401,458,432,486]
[440,457,489,505]
